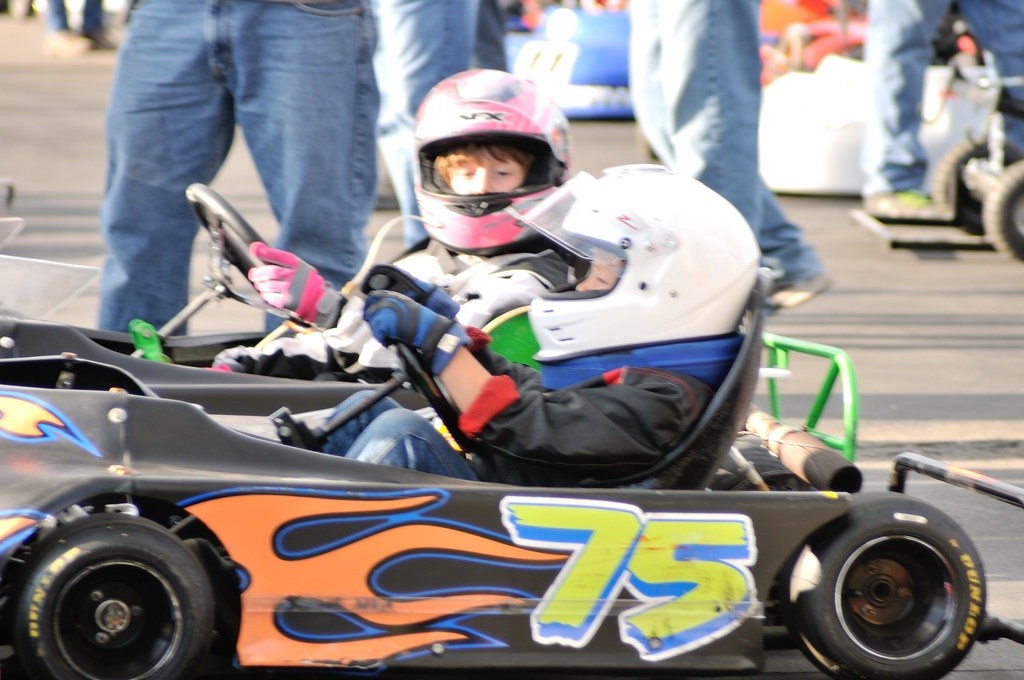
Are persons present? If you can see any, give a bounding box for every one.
[45,0,509,335]
[213,68,762,488]
[864,0,1024,199]
[629,0,831,307]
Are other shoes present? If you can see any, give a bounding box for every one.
[768,268,830,308]
[51,22,117,53]
[863,186,960,226]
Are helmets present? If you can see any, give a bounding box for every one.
[519,161,762,361]
[409,69,573,255]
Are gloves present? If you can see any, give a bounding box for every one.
[363,288,469,374]
[360,263,461,322]
[245,240,347,330]
